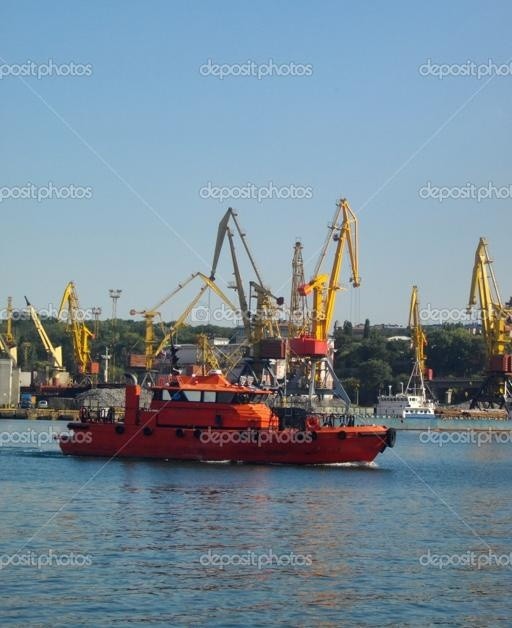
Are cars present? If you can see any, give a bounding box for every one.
[39,400,49,409]
[20,400,35,410]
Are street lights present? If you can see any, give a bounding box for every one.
[106,288,124,384]
[90,304,103,366]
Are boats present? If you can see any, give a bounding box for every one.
[54,368,397,466]
[372,380,437,419]
